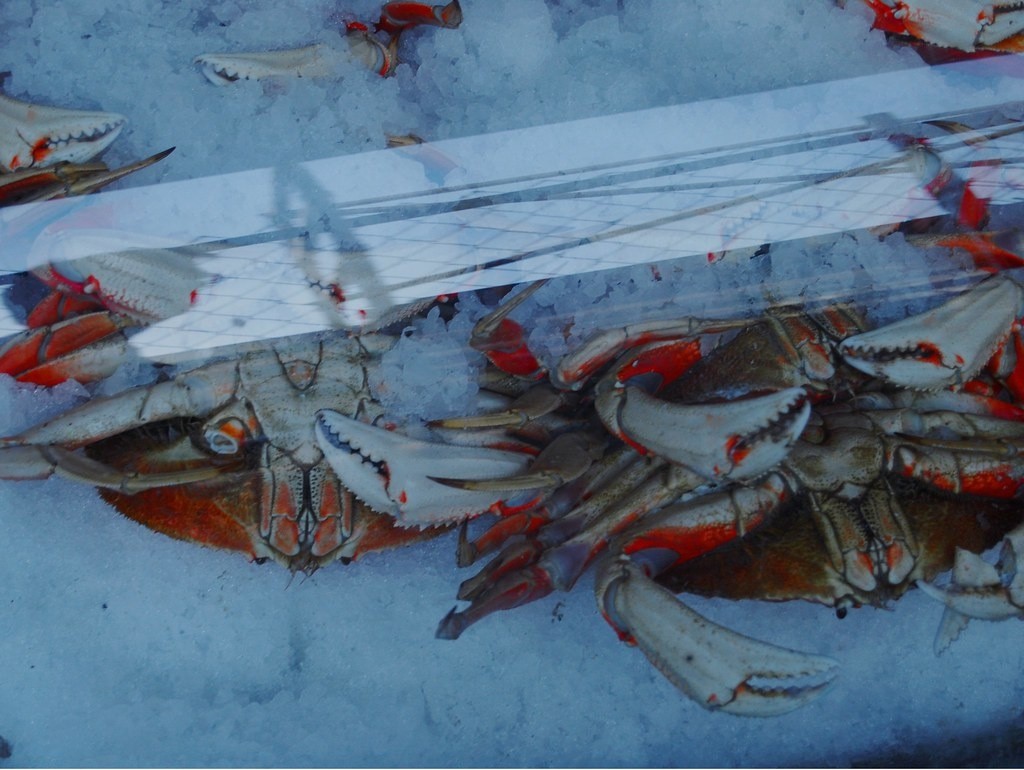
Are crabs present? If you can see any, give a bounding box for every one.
[0,0,1024,718]
